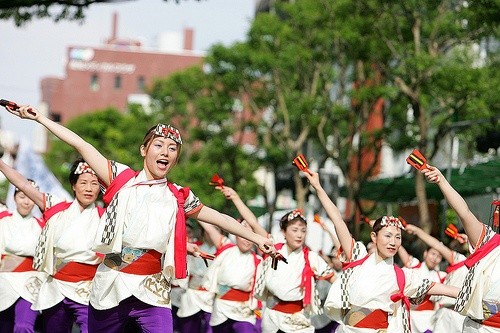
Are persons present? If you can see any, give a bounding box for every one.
[0,158,203,333]
[4,102,280,333]
[184,163,500,333]
[0,179,46,333]
